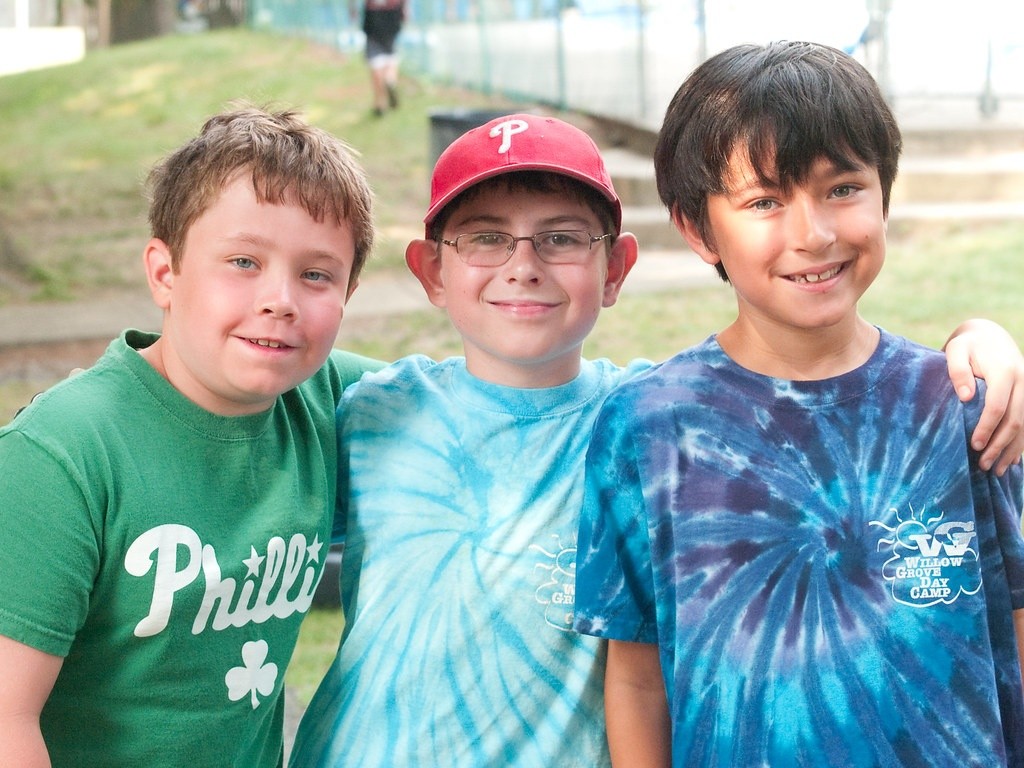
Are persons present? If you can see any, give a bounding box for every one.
[288,113,1023,768]
[577,41,1024,768]
[347,0,412,115]
[2,104,400,768]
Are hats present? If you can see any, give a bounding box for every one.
[423,112,623,246]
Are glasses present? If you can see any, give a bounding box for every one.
[431,229,614,267]
